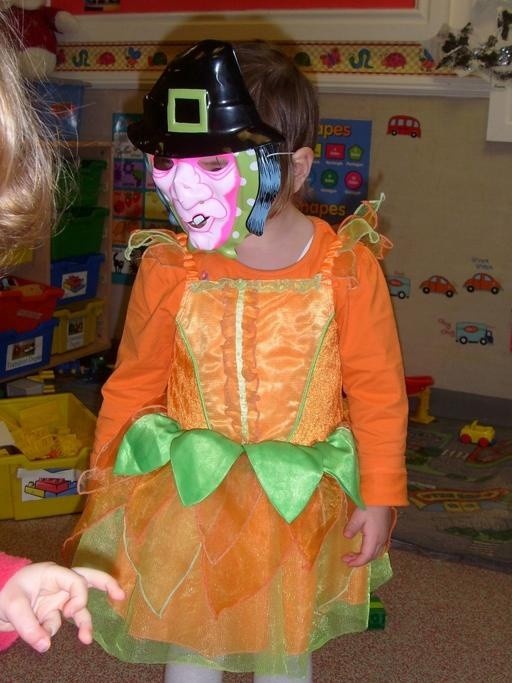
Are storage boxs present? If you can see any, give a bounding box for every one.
[0,158,110,381]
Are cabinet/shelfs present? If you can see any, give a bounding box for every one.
[0,140,112,385]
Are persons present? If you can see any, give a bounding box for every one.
[63,39,410,683]
[0,29,127,655]
